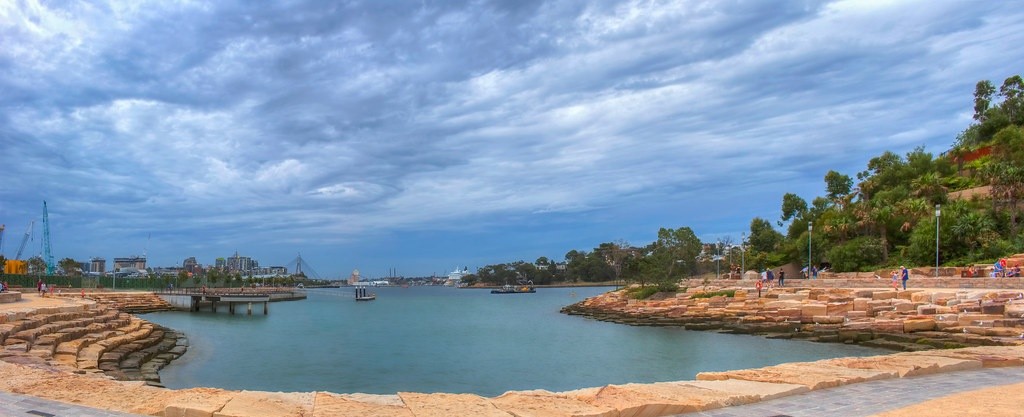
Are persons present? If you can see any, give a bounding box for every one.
[967,264,977,277]
[0,282,7,293]
[81,289,85,298]
[994,257,1021,277]
[38,279,53,297]
[240,284,244,292]
[761,267,785,289]
[203,285,206,295]
[729,264,740,274]
[799,265,818,279]
[893,266,908,291]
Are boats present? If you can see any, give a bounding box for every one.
[491,280,536,293]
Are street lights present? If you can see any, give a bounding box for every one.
[936,204,940,277]
[808,221,812,279]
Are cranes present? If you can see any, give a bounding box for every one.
[15,220,34,261]
[39,200,54,276]
